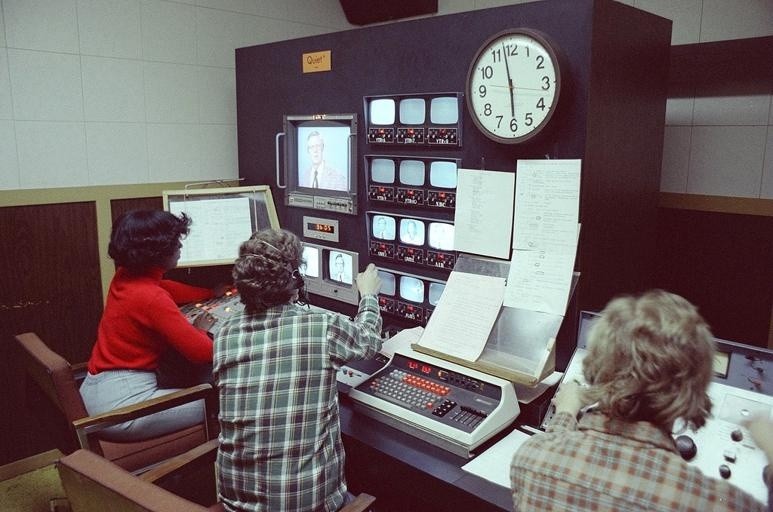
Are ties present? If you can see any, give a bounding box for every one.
[312,171,318,188]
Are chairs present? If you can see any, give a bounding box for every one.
[15,329,213,483]
[54,437,376,512]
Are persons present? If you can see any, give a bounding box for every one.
[209,227,384,512]
[298,131,347,190]
[79,208,231,444]
[332,254,350,283]
[510,286,773,512]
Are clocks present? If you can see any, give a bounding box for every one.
[463,26,568,150]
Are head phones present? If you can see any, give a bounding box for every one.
[236,240,305,294]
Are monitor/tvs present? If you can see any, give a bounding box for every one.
[429,159,457,191]
[369,156,397,185]
[397,96,427,126]
[297,244,321,279]
[398,275,427,304]
[427,281,446,307]
[162,186,282,270]
[398,158,427,188]
[321,248,354,289]
[366,97,396,127]
[377,270,397,298]
[397,218,426,249]
[429,96,458,126]
[285,115,354,198]
[371,213,397,241]
[426,221,456,255]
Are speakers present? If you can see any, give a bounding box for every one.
[340,1,439,27]
